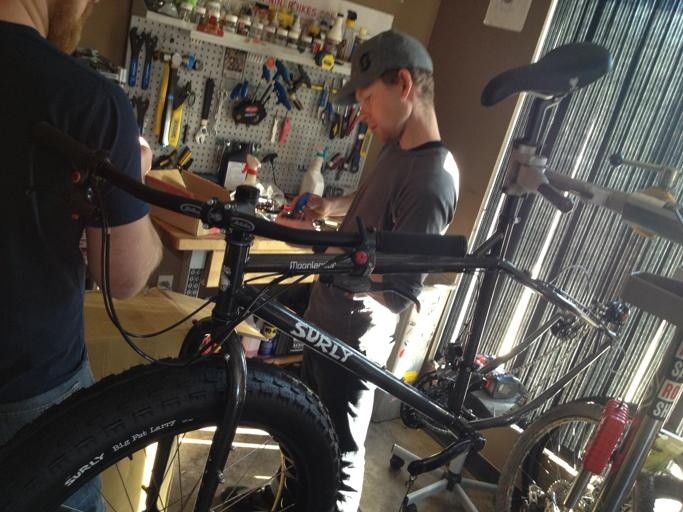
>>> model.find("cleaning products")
[296,145,332,200]
[230,152,262,203]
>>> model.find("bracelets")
[311,219,325,232]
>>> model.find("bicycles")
[0,37,639,510]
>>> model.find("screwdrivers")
[324,79,350,138]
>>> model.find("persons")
[220,28,462,512]
[2,0,158,510]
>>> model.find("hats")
[333,31,433,104]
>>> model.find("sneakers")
[222,484,278,511]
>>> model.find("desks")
[151,216,347,367]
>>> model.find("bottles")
[179,0,368,62]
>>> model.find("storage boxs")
[84,286,269,512]
[144,168,231,238]
